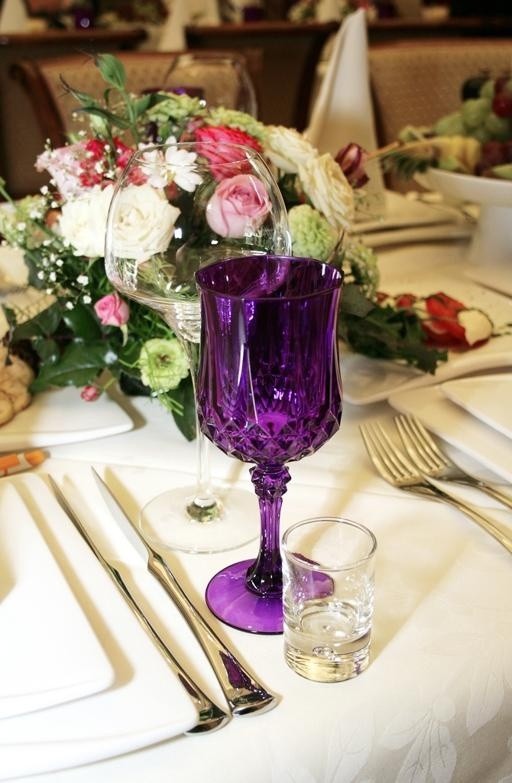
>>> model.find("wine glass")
[192,252,347,637]
[100,139,296,560]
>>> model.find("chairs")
[182,22,341,134]
[298,23,511,147]
[15,45,257,151]
[2,28,148,198]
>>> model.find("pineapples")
[378,135,482,184]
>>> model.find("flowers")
[0,56,499,441]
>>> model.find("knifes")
[90,467,274,716]
[39,469,232,734]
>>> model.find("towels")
[297,7,386,221]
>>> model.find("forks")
[356,407,512,554]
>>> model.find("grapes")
[434,76,512,165]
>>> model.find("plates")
[342,187,474,247]
[386,369,512,490]
[340,275,512,409]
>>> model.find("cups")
[277,514,380,684]
[158,49,261,125]
